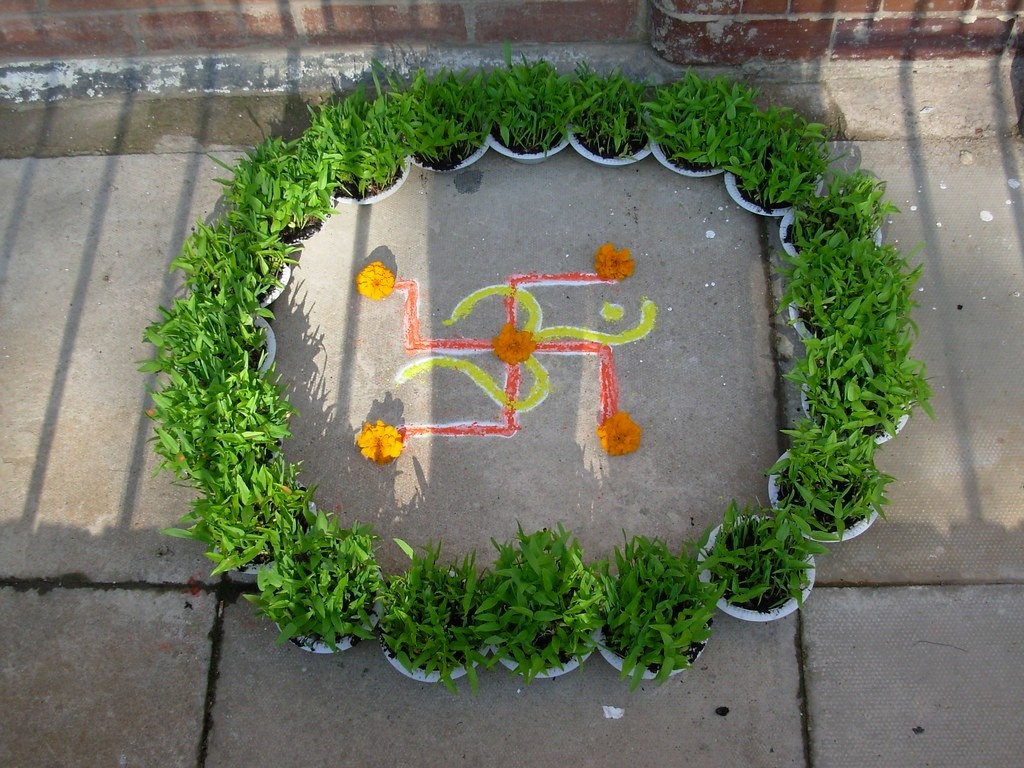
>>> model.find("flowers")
[492,322,538,366]
[593,242,635,283]
[354,418,403,465]
[596,410,640,457]
[354,261,396,302]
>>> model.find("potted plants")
[132,31,935,577]
[694,496,831,624]
[242,502,383,656]
[376,534,489,695]
[594,526,729,693]
[469,518,604,688]
